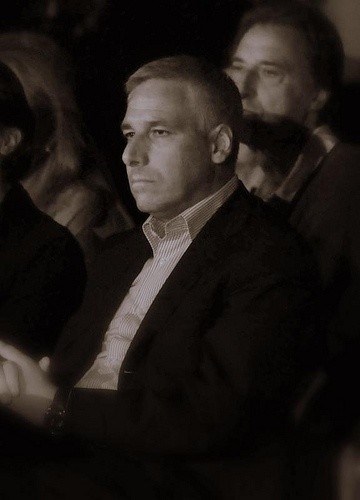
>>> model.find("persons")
[219,6,359,346]
[0,64,85,499]
[0,56,328,500]
[3,30,126,280]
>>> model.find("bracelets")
[41,385,71,436]
